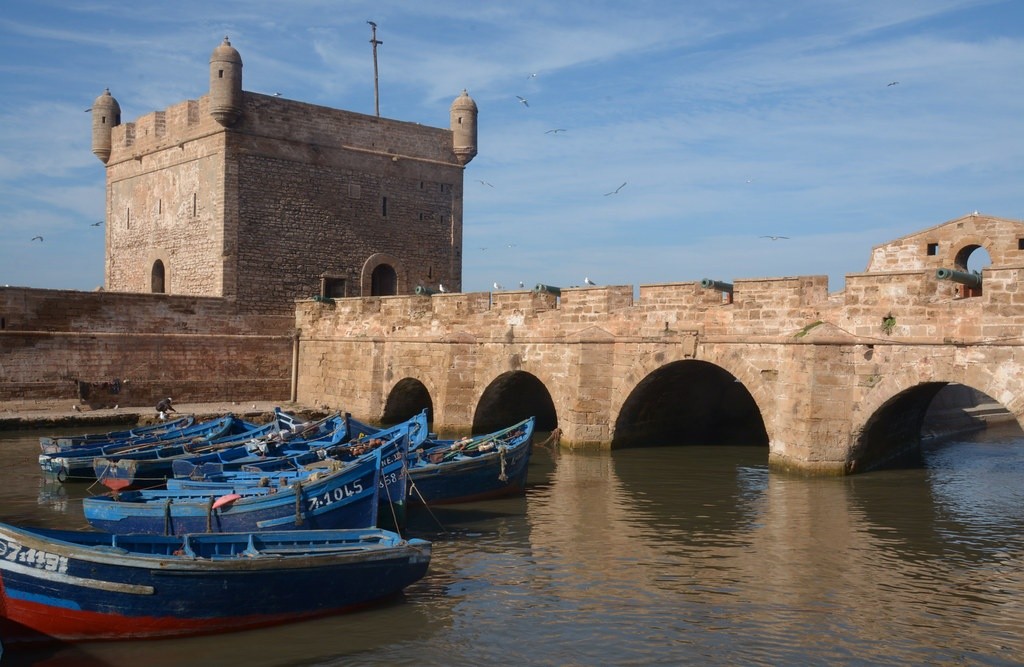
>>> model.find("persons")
[156,397,176,414]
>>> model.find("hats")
[168,398,172,403]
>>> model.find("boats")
[82,448,381,536]
[166,426,410,518]
[37,406,536,499]
[0,520,433,641]
